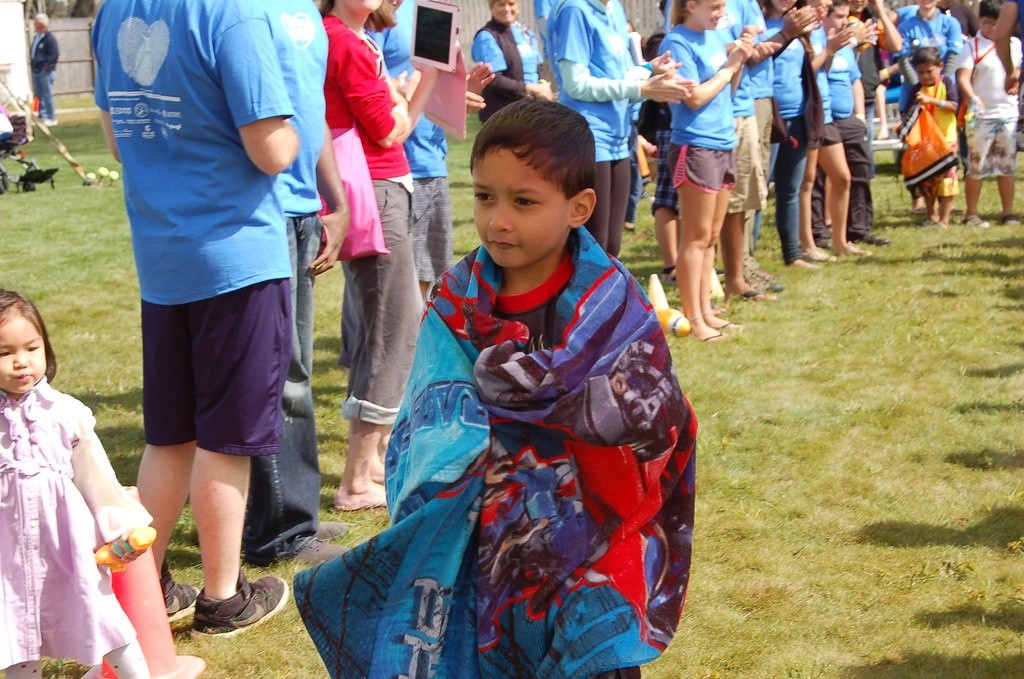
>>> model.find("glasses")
[980,17,996,28]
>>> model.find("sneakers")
[190,570,290,637]
[285,537,351,566]
[314,520,348,537]
[154,559,198,623]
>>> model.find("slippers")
[741,289,776,302]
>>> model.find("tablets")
[410,0,460,72]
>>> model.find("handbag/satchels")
[317,127,391,260]
[898,103,958,190]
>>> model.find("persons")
[92,0,300,638]
[0,288,153,679]
[30,12,59,127]
[317,0,496,511]
[470,0,554,122]
[238,0,351,566]
[294,95,699,679]
[532,0,1024,341]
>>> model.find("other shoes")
[335,482,388,511]
[920,218,947,229]
[641,190,655,202]
[661,264,677,280]
[800,247,837,263]
[961,214,990,229]
[790,259,817,270]
[687,315,726,342]
[704,314,740,333]
[1000,213,1020,225]
[837,241,869,258]
[746,270,781,292]
[625,221,635,232]
[852,231,890,248]
[43,119,59,127]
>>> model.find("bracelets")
[939,100,946,109]
[929,98,934,103]
[967,96,979,105]
[639,62,654,76]
[718,70,732,82]
[849,37,859,47]
[882,67,889,80]
[779,31,788,43]
[530,84,536,92]
